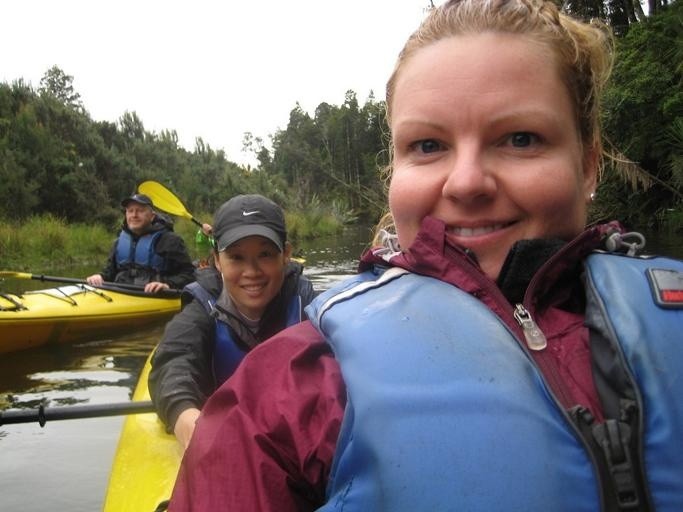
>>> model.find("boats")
[0,253,307,355]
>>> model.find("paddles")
[137,180,213,233]
[0,270,182,296]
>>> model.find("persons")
[164,0,682,512]
[84,192,196,297]
[146,191,332,453]
[195,221,215,260]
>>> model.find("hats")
[211,192,286,255]
[119,193,153,208]
